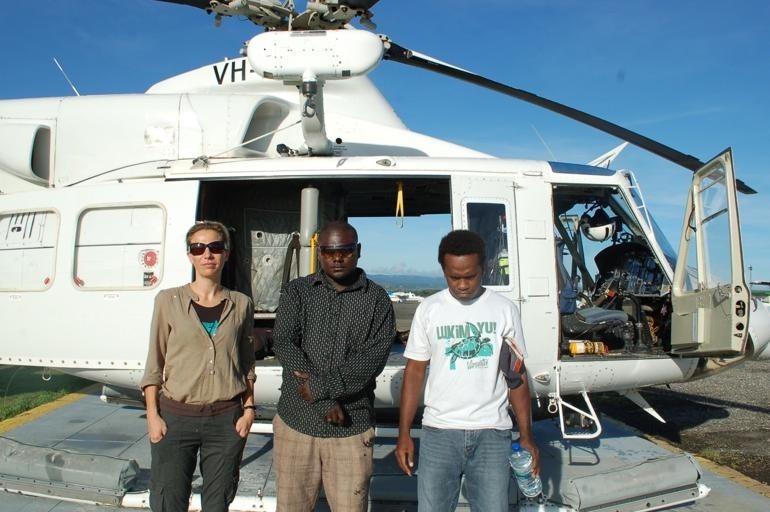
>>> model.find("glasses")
[190,241,225,255]
[319,248,355,258]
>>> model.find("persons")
[394,229,541,512]
[141,219,256,512]
[273,222,396,512]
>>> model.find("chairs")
[553,237,633,356]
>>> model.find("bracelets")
[243,405,256,410]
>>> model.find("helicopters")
[1,0,769,443]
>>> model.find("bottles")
[569,340,609,355]
[507,441,543,498]
[498,248,510,276]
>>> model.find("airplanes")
[388,291,427,303]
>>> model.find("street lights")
[748,261,753,286]
